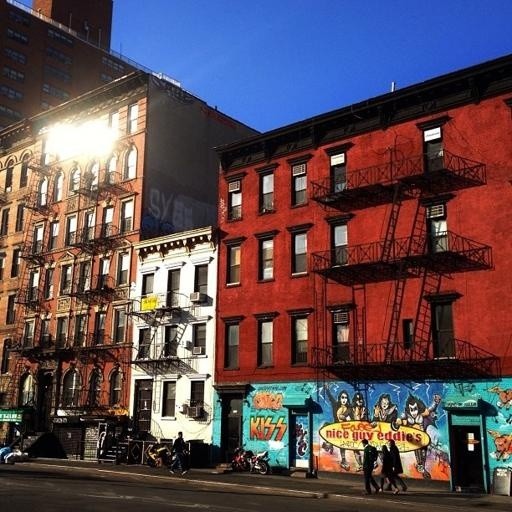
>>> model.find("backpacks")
[366,446,378,461]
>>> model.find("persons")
[377,444,399,495]
[350,391,377,470]
[373,392,401,432]
[323,382,353,470]
[386,440,408,492]
[168,430,189,475]
[393,391,442,473]
[362,439,382,496]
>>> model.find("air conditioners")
[185,406,201,418]
[192,346,205,355]
[189,292,207,303]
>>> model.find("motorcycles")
[232,444,271,475]
[145,441,170,468]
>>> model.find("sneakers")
[364,485,408,495]
[169,470,187,476]
